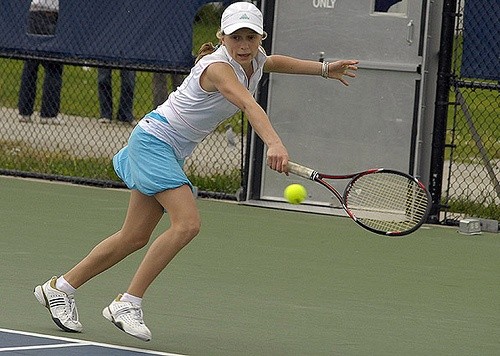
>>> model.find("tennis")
[284,184,307,205]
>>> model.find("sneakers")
[102,293,152,343]
[33,275,83,334]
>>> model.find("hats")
[220,1,264,36]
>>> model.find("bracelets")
[321,60,329,79]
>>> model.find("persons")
[32,1,359,342]
